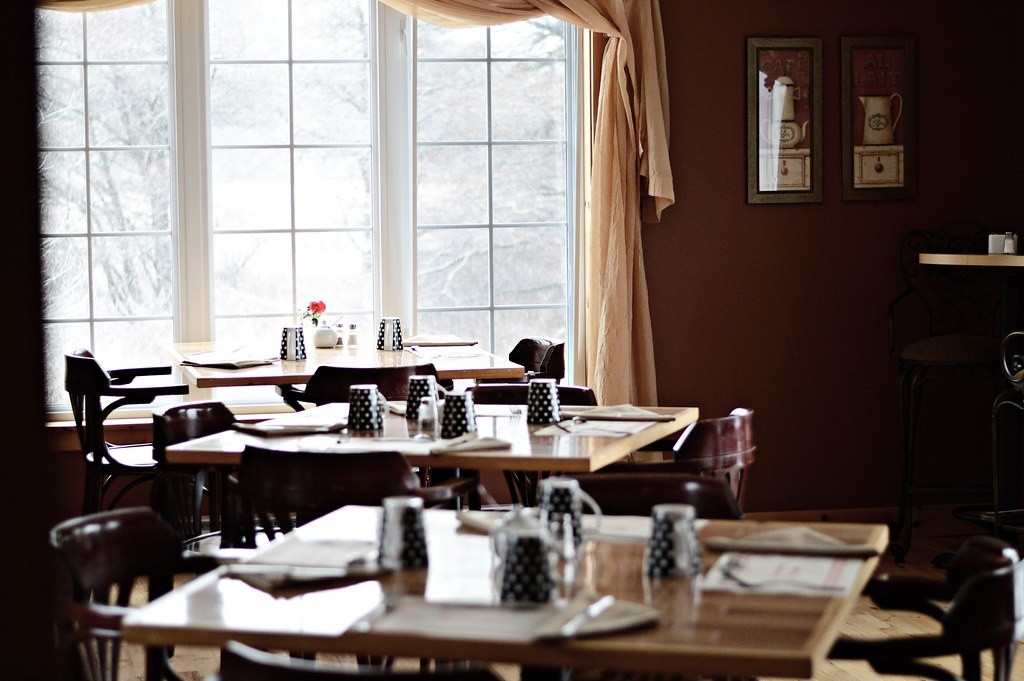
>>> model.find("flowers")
[302,300,326,325]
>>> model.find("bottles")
[348,324,357,349]
[1003,232,1014,254]
[335,324,344,347]
[419,397,438,435]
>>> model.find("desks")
[173,337,525,412]
[164,400,701,515]
[120,502,891,678]
[918,252,1024,538]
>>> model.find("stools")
[883,220,1019,566]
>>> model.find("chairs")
[46,335,1021,681]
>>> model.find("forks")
[554,422,630,434]
[509,408,522,413]
[721,568,843,590]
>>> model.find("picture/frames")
[839,35,919,202]
[745,37,824,205]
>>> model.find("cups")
[377,497,429,571]
[526,378,560,423]
[646,504,702,580]
[406,375,448,420]
[988,235,1017,254]
[537,478,601,545]
[377,317,403,351]
[494,529,581,607]
[442,392,476,439]
[347,384,389,431]
[280,325,306,361]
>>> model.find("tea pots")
[313,320,338,348]
